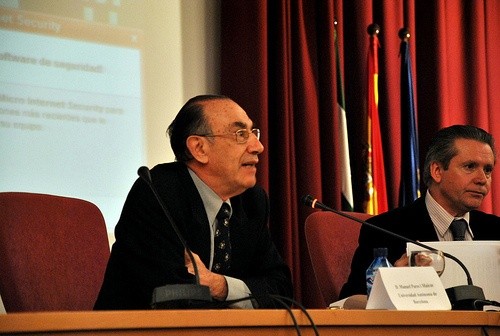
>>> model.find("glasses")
[198,129,260,143]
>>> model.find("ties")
[211,202,233,276]
[449,219,467,241]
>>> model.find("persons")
[93,94,298,311]
[339,124,500,300]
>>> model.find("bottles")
[366,247,395,297]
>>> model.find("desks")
[0,309,500,336]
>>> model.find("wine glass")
[410,250,445,277]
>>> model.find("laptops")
[406,240,500,303]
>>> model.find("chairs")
[305,211,376,308]
[0,193,110,313]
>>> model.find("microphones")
[301,194,485,310]
[137,166,212,309]
